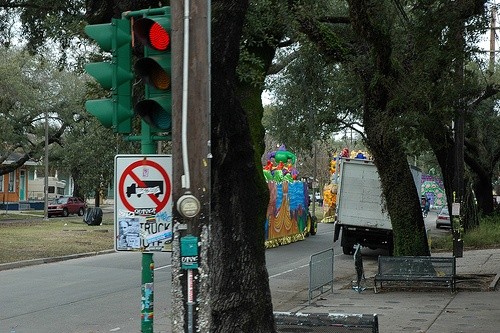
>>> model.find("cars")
[48,196,87,216]
[436,206,451,228]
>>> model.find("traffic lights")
[150,22,171,130]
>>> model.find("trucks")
[334,156,422,259]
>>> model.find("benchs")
[373,254,456,295]
